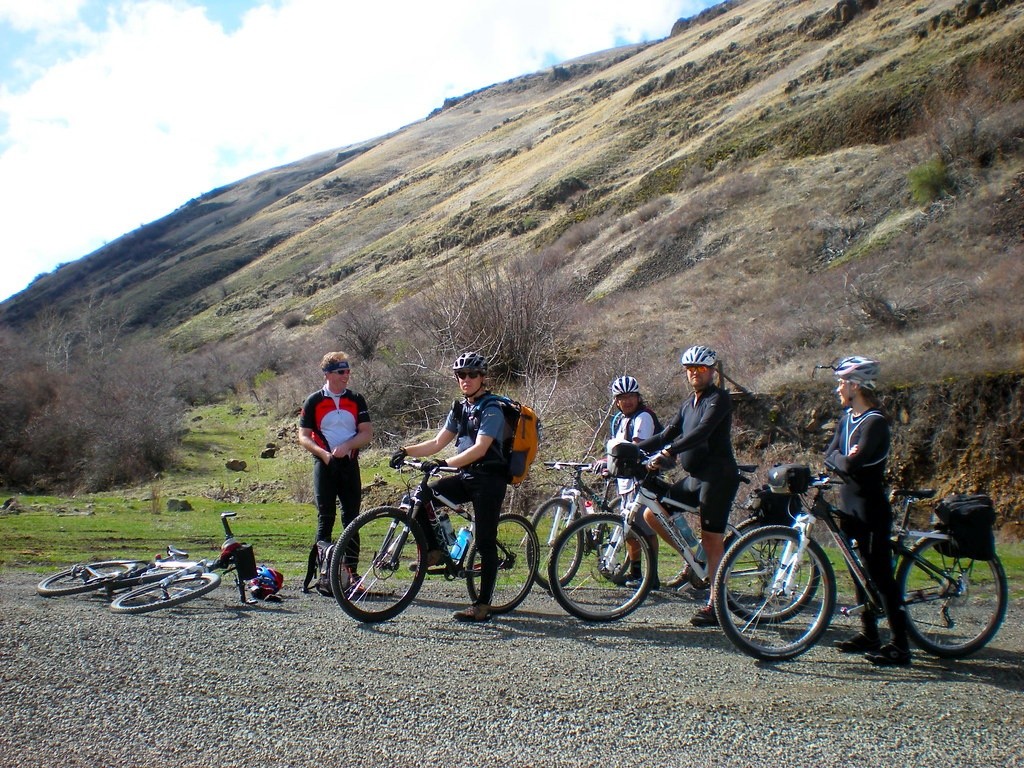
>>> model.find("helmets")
[245,566,283,598]
[835,356,881,389]
[453,352,488,374]
[611,376,639,397]
[681,345,718,368]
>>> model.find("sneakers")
[866,644,911,668]
[407,551,443,570]
[452,604,492,622]
[833,633,882,653]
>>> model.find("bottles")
[851,538,864,568]
[585,500,595,515]
[672,511,698,547]
[439,509,458,546]
[450,526,471,560]
[695,542,706,561]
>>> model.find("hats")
[322,360,350,374]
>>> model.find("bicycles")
[329,458,540,624]
[527,462,635,589]
[713,459,1008,661]
[37,512,258,614]
[547,438,821,623]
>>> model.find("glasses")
[456,372,480,379]
[685,366,709,373]
[331,370,351,374]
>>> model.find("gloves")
[420,459,448,475]
[646,453,669,471]
[389,448,407,469]
[593,459,607,474]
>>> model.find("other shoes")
[666,568,688,587]
[613,561,643,584]
[692,605,719,627]
[349,576,365,591]
[626,576,661,591]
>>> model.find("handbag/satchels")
[932,495,995,561]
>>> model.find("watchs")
[664,444,673,453]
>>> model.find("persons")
[390,352,507,620]
[592,374,661,589]
[298,352,372,593]
[637,346,740,627]
[823,356,911,666]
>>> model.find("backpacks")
[457,395,541,487]
[302,539,351,598]
